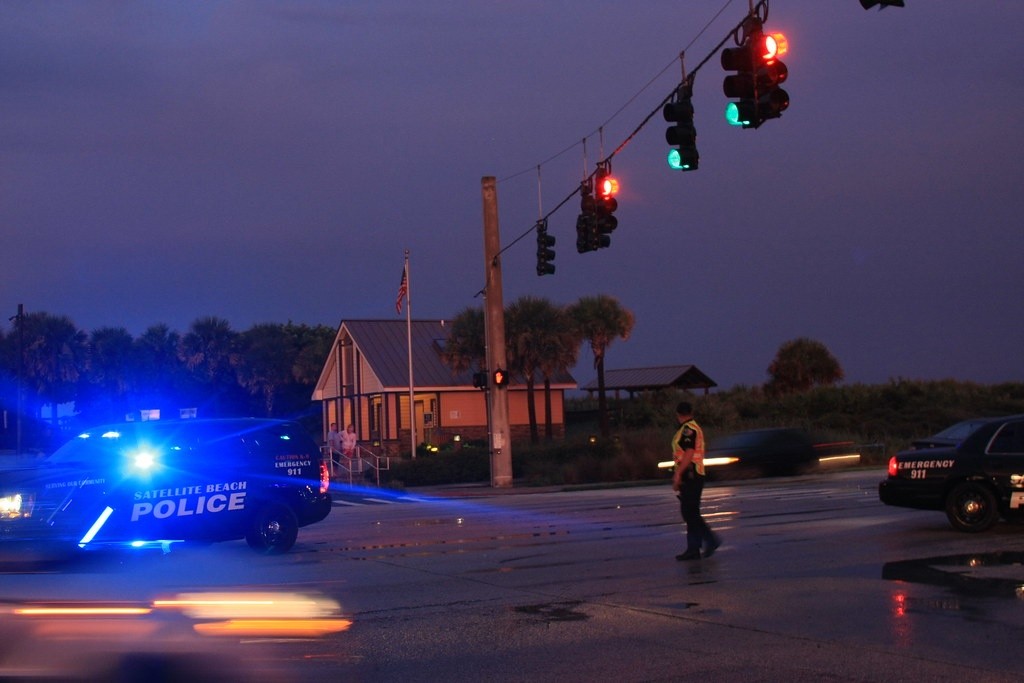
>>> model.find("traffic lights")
[596,164,618,248]
[577,193,597,253]
[751,31,788,118]
[721,42,767,129]
[536,225,555,276]
[662,89,699,172]
[494,368,510,386]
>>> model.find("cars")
[656,426,817,484]
[877,415,1024,535]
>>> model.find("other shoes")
[701,538,722,558]
[675,547,701,560]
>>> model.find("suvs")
[0,417,332,572]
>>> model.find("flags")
[396,263,407,314]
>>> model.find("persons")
[338,424,356,474]
[672,402,722,560]
[327,423,341,469]
